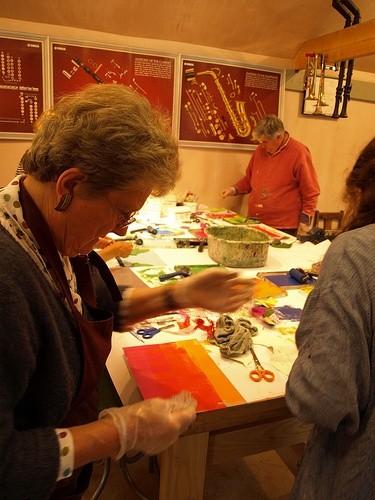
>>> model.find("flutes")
[330,0,351,119]
[341,0,365,121]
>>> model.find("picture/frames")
[0,30,45,140]
[176,53,286,151]
[46,35,178,143]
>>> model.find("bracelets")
[164,280,177,310]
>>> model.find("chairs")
[314,210,344,230]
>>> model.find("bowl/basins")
[202,225,274,268]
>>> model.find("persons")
[219,113,320,243]
[283,137,375,500]
[0,83,257,500]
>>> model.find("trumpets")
[311,71,324,116]
[305,54,317,101]
[316,58,329,107]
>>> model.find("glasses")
[104,193,137,230]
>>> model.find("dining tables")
[95,203,323,500]
